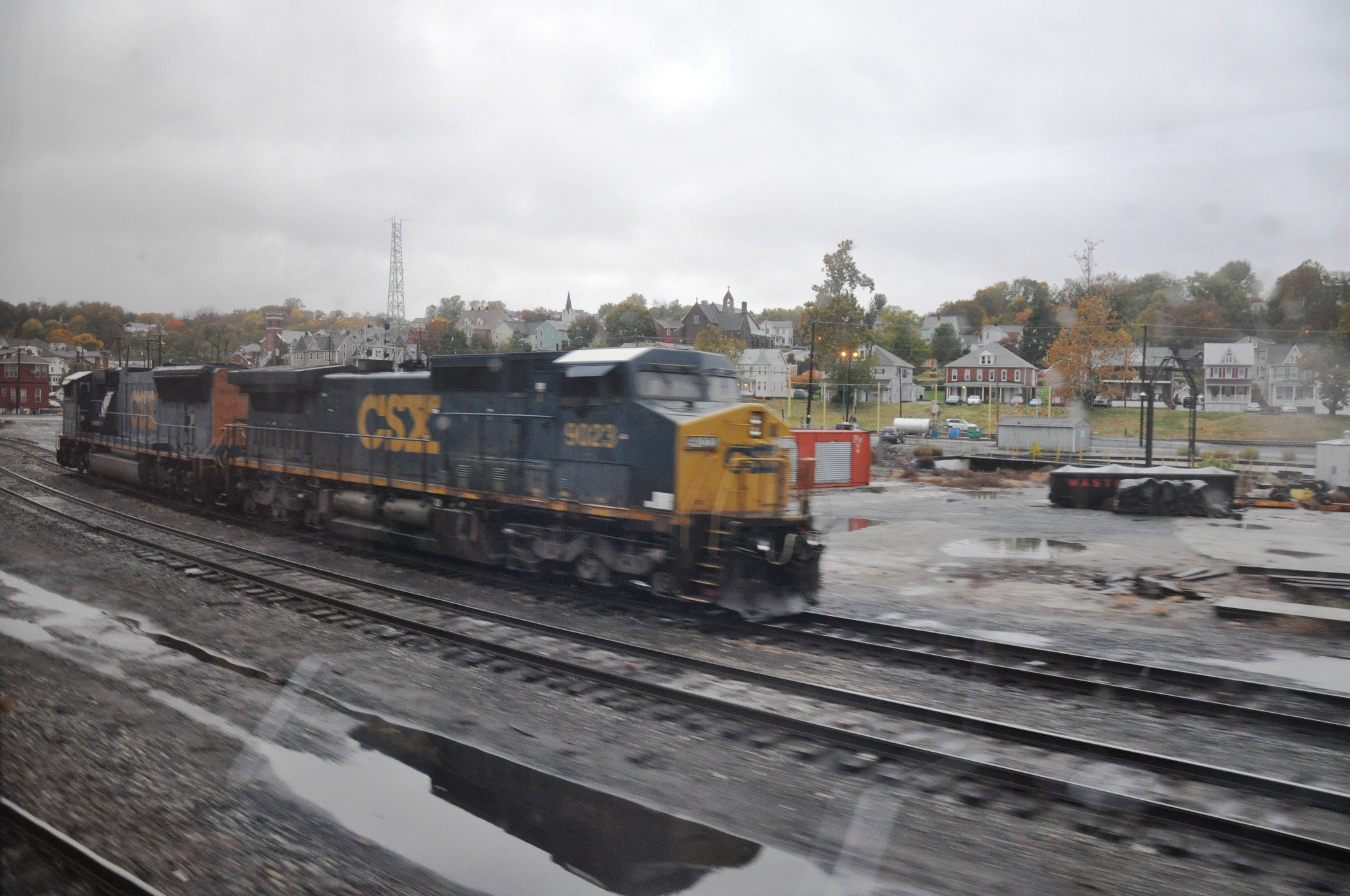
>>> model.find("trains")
[57,347,821,622]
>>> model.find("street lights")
[841,347,858,421]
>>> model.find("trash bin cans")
[835,422,853,431]
[967,427,983,438]
[951,427,961,438]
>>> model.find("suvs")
[1182,397,1197,408]
[945,418,979,432]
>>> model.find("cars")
[1245,402,1262,413]
[946,394,1113,408]
[1281,403,1297,413]
